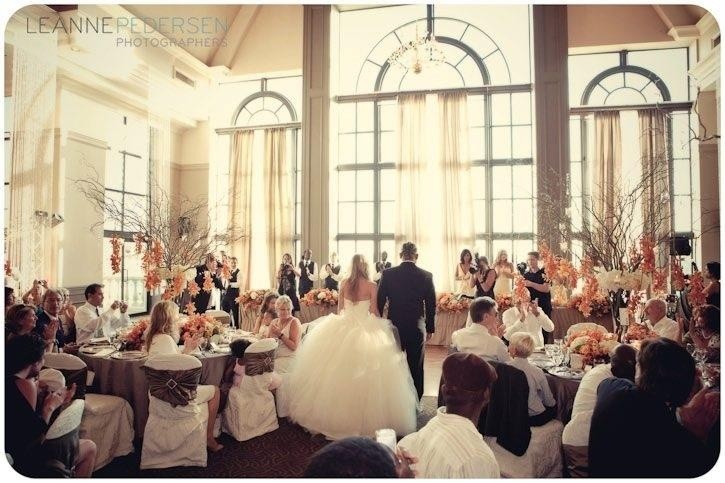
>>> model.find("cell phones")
[375,429,398,450]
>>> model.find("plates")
[82,347,115,354]
[214,347,231,353]
[88,341,110,345]
[110,351,145,362]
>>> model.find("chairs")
[476,360,564,478]
[206,310,230,327]
[41,353,135,471]
[276,323,313,423]
[140,354,209,471]
[222,338,279,442]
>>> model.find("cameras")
[36,280,49,288]
[468,253,482,275]
[280,264,288,271]
[216,261,223,268]
[516,262,528,272]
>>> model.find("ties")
[306,262,308,267]
[332,263,334,268]
[96,308,100,317]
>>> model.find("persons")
[285,254,420,441]
[395,353,501,478]
[375,251,391,285]
[302,435,416,478]
[452,243,720,479]
[5,250,342,478]
[377,242,436,413]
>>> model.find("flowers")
[177,313,226,345]
[495,292,515,312]
[437,294,470,314]
[567,323,618,369]
[566,292,609,319]
[625,325,659,349]
[301,289,339,312]
[235,289,269,313]
[117,319,149,351]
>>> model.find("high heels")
[211,444,223,452]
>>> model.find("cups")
[545,337,565,369]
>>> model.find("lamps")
[385,22,446,74]
